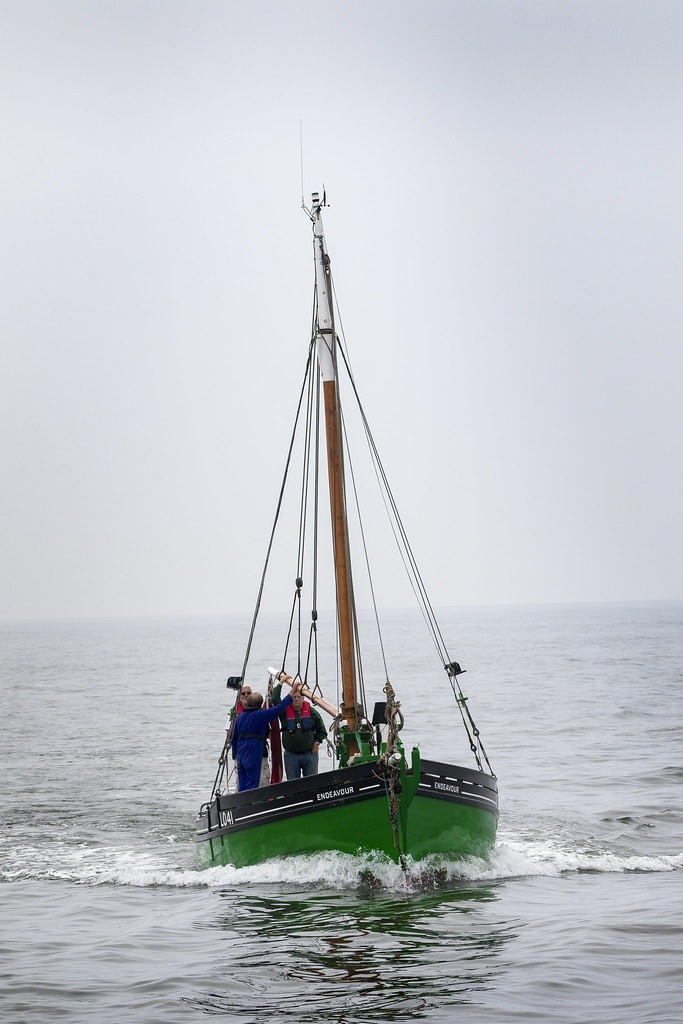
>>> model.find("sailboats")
[196,187,499,878]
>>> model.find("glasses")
[240,692,251,696]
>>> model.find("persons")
[232,684,304,792]
[270,676,327,781]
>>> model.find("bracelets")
[315,740,319,744]
